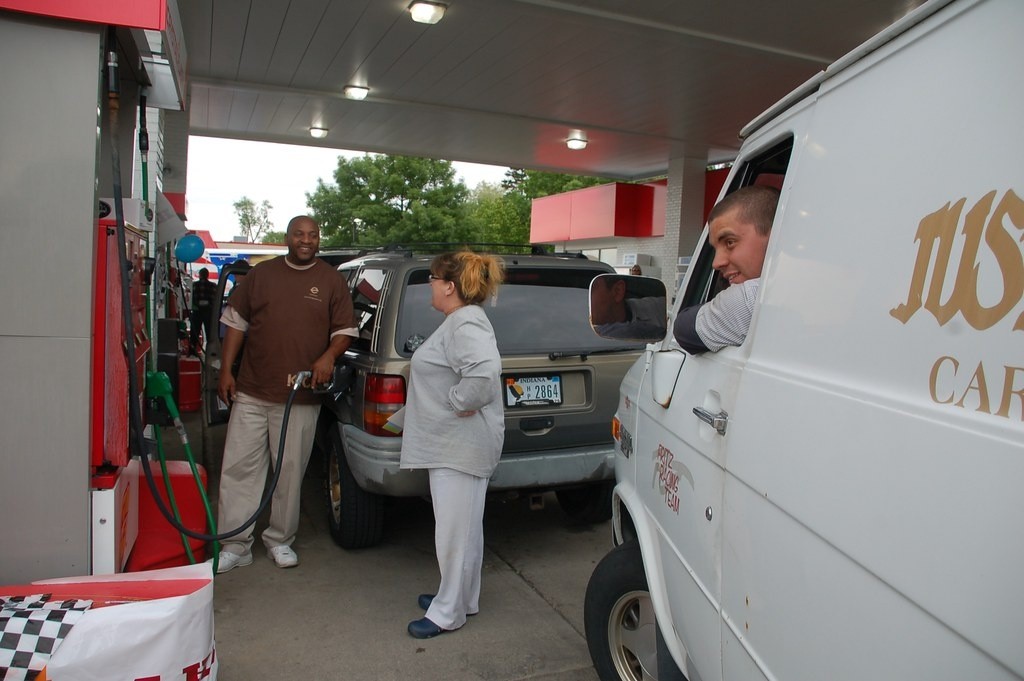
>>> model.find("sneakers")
[266,545,299,568]
[202,551,253,573]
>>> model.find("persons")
[204,216,360,573]
[591,275,668,340]
[631,265,642,275]
[188,266,217,350]
[670,181,781,355]
[398,251,505,638]
[227,260,250,298]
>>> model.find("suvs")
[203,243,646,551]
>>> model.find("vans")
[583,0,1024,681]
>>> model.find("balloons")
[174,234,205,263]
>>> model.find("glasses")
[428,275,449,286]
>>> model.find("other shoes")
[408,616,443,638]
[419,593,434,609]
